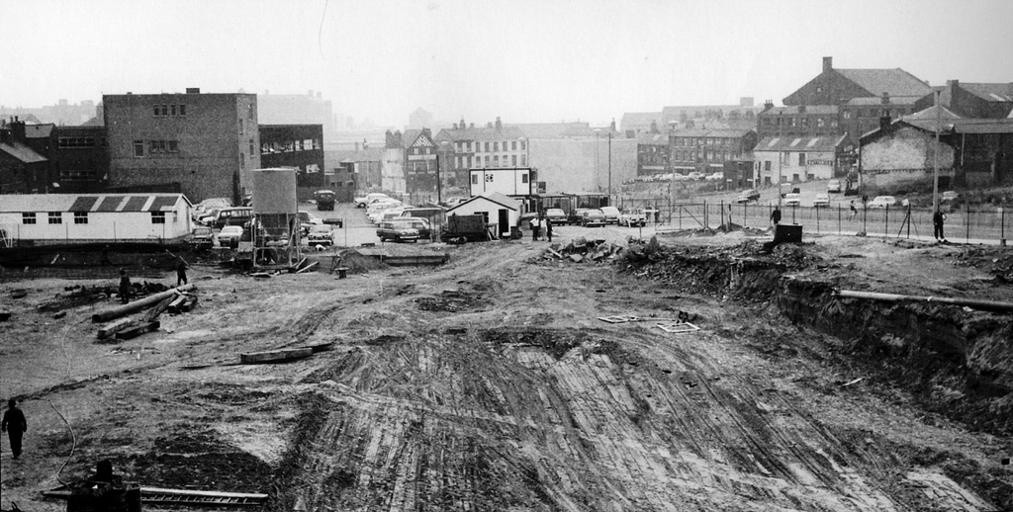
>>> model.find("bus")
[313,190,336,210]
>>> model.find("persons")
[119,266,130,304]
[177,261,188,285]
[934,206,944,239]
[530,215,555,242]
[770,205,782,225]
[2,398,28,460]
[850,200,858,214]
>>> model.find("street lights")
[594,129,602,164]
[776,106,789,210]
[668,121,678,223]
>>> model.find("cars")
[544,206,646,227]
[301,211,343,246]
[940,191,959,205]
[738,189,760,203]
[781,180,896,210]
[190,207,253,248]
[637,172,722,182]
[353,193,439,242]
